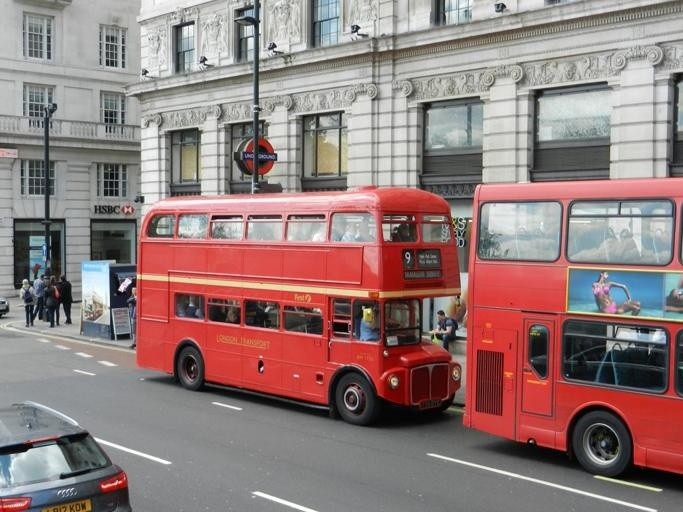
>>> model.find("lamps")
[351,25,369,38]
[268,42,283,54]
[141,69,154,78]
[493,2,507,13]
[199,57,215,67]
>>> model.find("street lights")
[41,103,57,279]
[235,15,260,196]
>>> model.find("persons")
[248,216,418,244]
[663,276,682,312]
[31,272,45,321]
[426,308,456,351]
[19,278,35,327]
[43,274,59,328]
[357,306,379,342]
[589,270,641,317]
[125,286,137,349]
[184,295,203,319]
[56,274,72,324]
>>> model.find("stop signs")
[241,137,277,174]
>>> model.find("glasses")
[675,291,682,300]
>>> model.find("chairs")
[515,225,668,265]
[530,329,683,394]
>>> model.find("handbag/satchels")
[54,289,60,299]
[431,330,442,346]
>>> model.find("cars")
[0,296,9,318]
[0,401,133,511]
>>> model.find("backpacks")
[22,287,33,303]
[444,317,457,329]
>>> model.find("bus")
[134,186,460,426]
[465,177,683,479]
